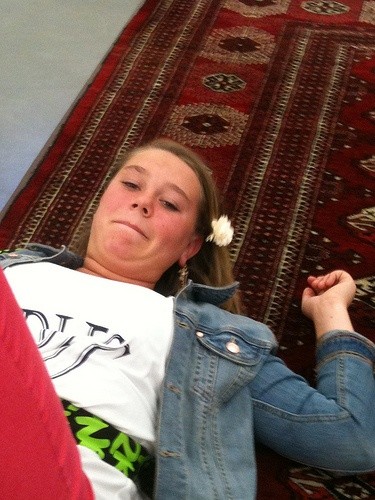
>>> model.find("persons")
[1,135,375,500]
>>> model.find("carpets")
[0,0,375,500]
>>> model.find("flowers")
[206,213,234,246]
